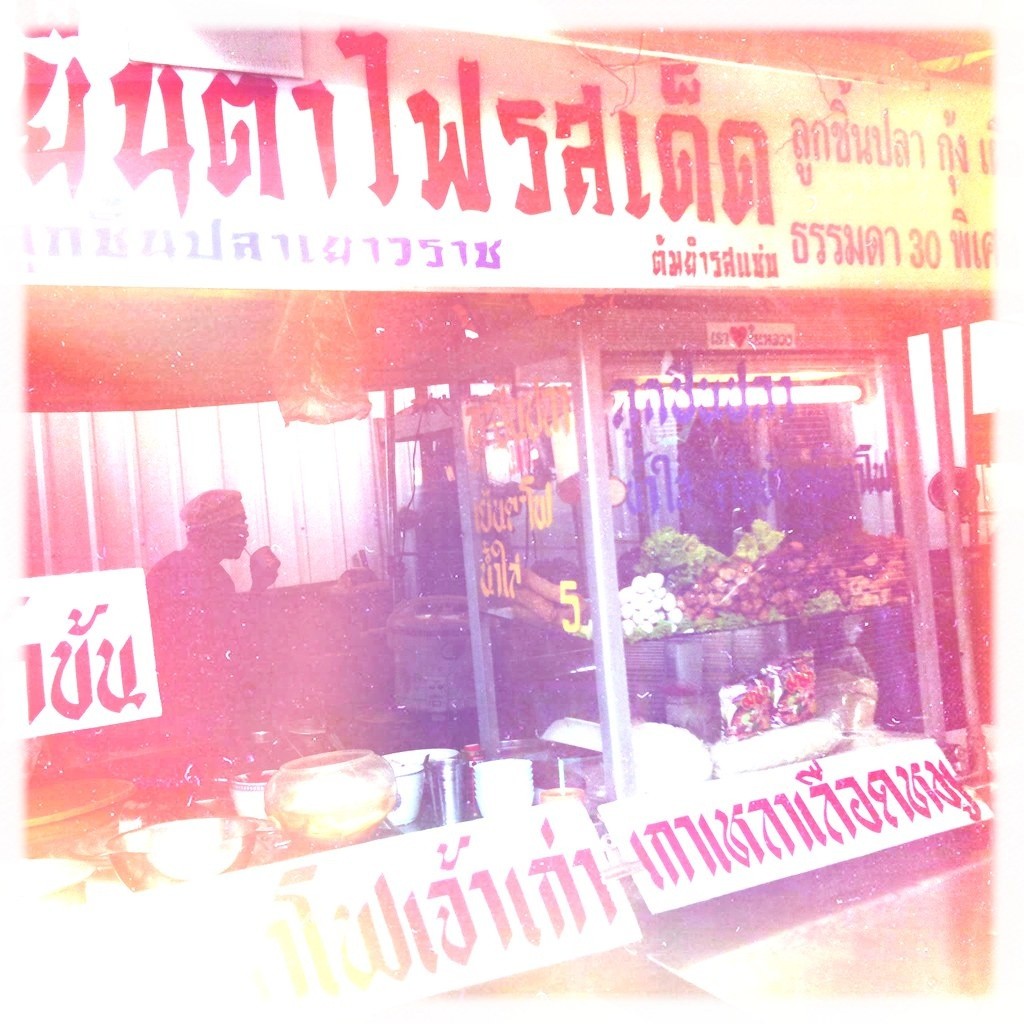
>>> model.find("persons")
[143,488,281,731]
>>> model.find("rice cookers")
[387,595,476,713]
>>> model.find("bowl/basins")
[105,816,259,893]
[541,717,602,752]
[473,759,535,818]
[26,777,137,859]
[384,762,425,827]
[230,767,279,820]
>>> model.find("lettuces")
[619,518,843,643]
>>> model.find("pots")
[264,748,398,843]
[384,749,464,833]
